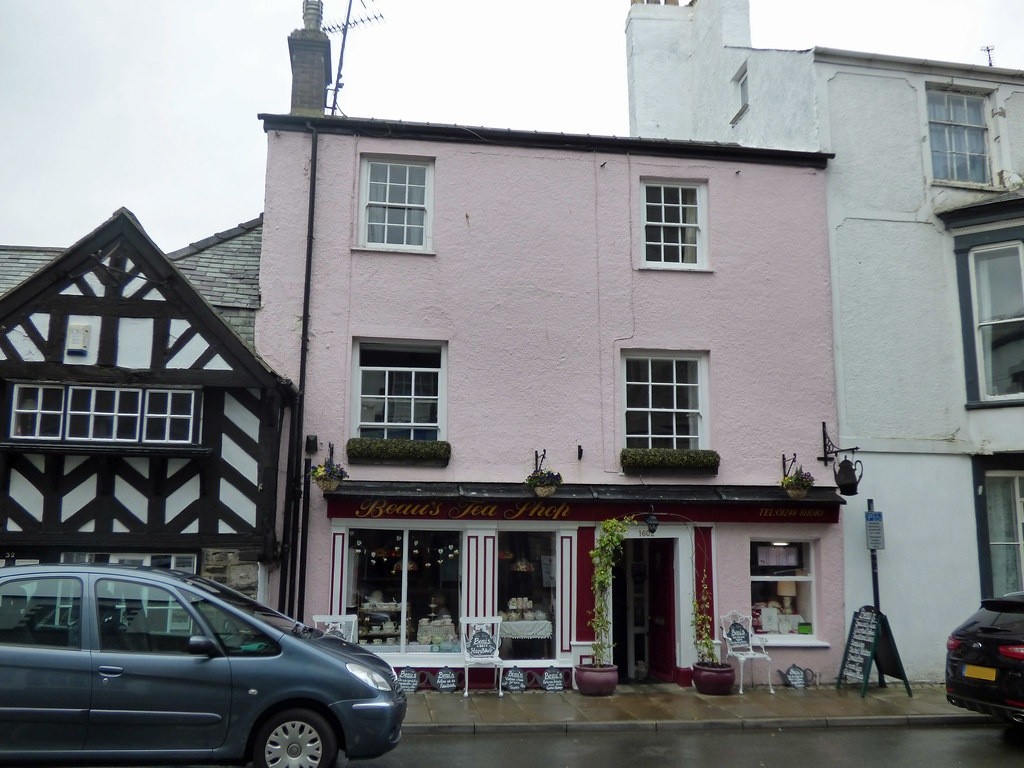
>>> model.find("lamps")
[645,504,660,534]
[778,581,797,615]
[817,421,864,497]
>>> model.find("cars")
[0,561,406,768]
[946,591,1024,727]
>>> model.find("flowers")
[306,457,350,481]
[521,466,563,486]
[780,464,817,486]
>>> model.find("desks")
[502,621,552,658]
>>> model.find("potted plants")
[690,569,737,696]
[574,514,638,696]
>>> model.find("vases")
[535,486,557,498]
[786,486,807,497]
[316,480,339,491]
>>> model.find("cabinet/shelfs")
[359,606,411,642]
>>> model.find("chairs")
[721,609,776,695]
